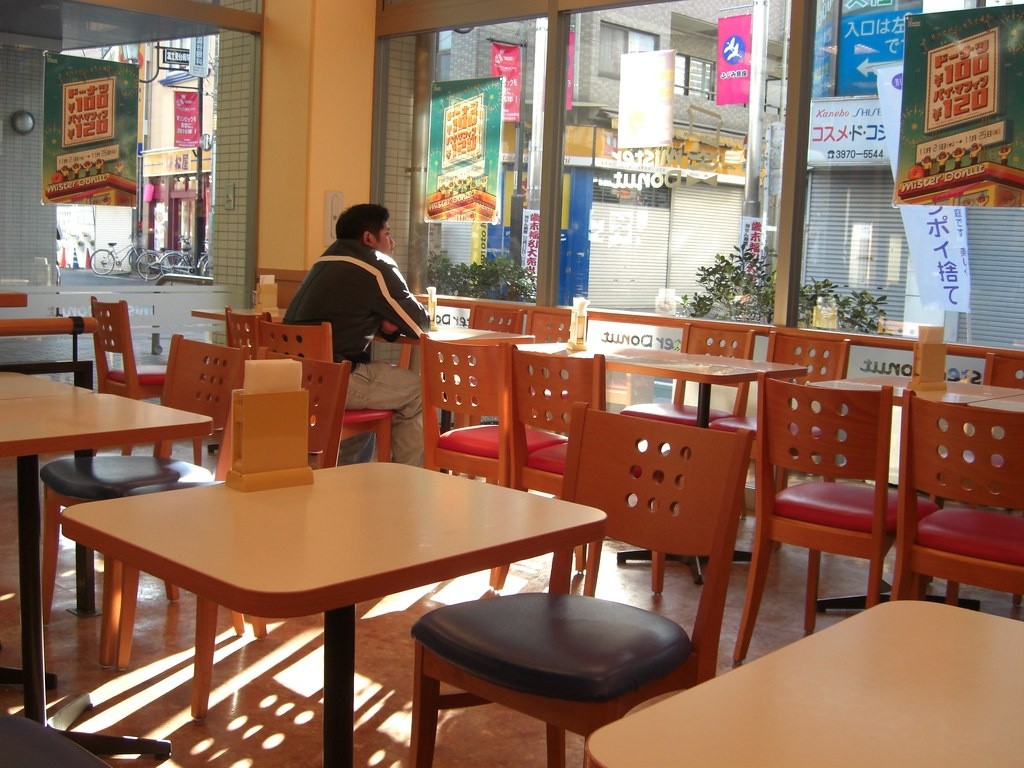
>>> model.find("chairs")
[731,369,939,662]
[422,334,588,586]
[526,307,589,401]
[931,352,1024,511]
[452,305,524,481]
[621,322,756,519]
[41,295,393,671]
[710,330,852,551]
[493,345,674,595]
[408,401,757,768]
[892,391,1024,604]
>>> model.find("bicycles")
[89,236,149,275]
[136,236,209,281]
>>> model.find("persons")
[281,205,430,469]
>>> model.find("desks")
[801,373,1024,613]
[191,305,809,588]
[60,463,609,768]
[0,372,215,760]
[585,601,1024,768]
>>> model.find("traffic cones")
[60,246,67,268]
[86,247,91,270]
[72,248,84,269]
